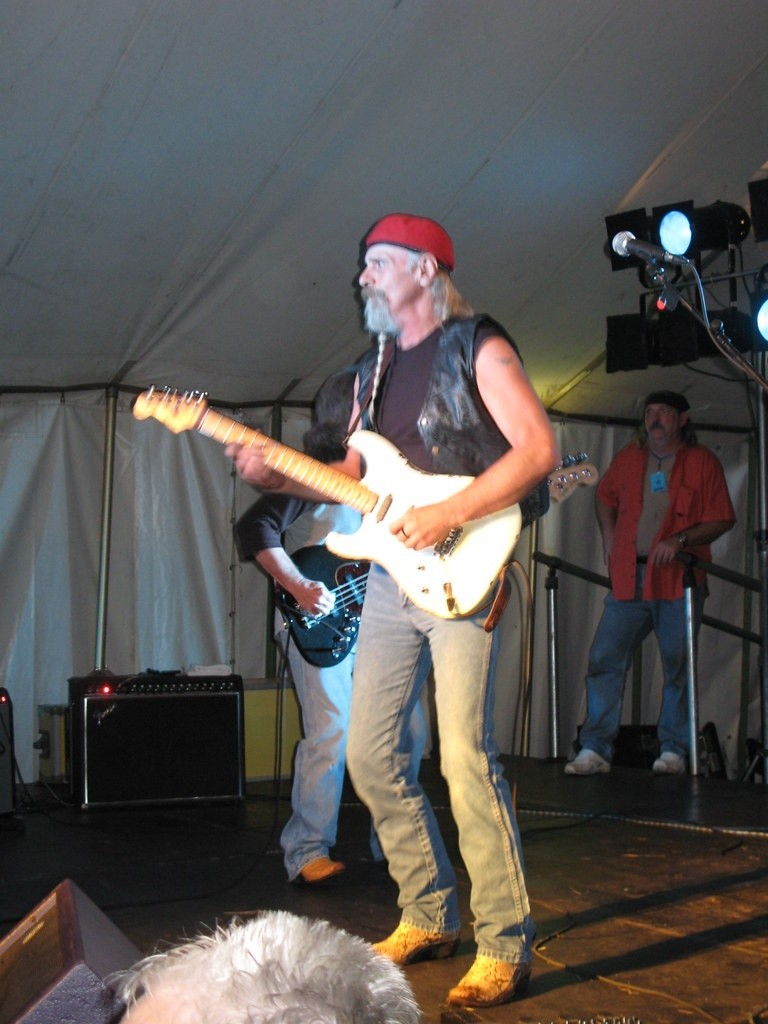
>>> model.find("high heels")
[371,921,463,966]
[446,955,532,1007]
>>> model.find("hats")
[644,390,691,414]
[365,214,457,273]
[316,370,357,423]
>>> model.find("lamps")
[605,178,768,373]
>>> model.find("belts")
[636,555,648,564]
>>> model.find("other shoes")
[380,859,388,865]
[301,856,345,883]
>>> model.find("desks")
[241,677,304,782]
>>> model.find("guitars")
[272,450,599,669]
[132,384,522,620]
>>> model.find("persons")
[565,389,736,776]
[233,368,428,882]
[225,213,561,1006]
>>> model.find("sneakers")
[564,748,611,774]
[653,751,686,773]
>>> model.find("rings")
[402,528,408,538]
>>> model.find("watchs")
[677,532,687,546]
